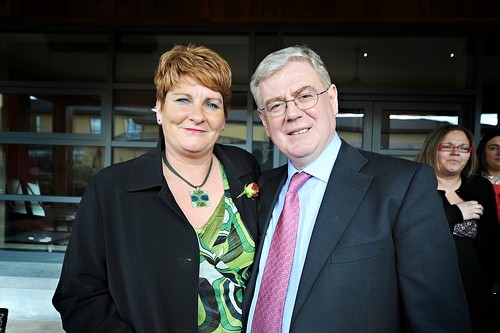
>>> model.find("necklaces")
[163,152,214,208]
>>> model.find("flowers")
[236,183,260,200]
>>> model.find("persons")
[240,46,466,333]
[419,124,500,333]
[52,43,261,333]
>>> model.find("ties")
[251,171,313,333]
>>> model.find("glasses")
[259,88,328,117]
[437,143,474,153]
